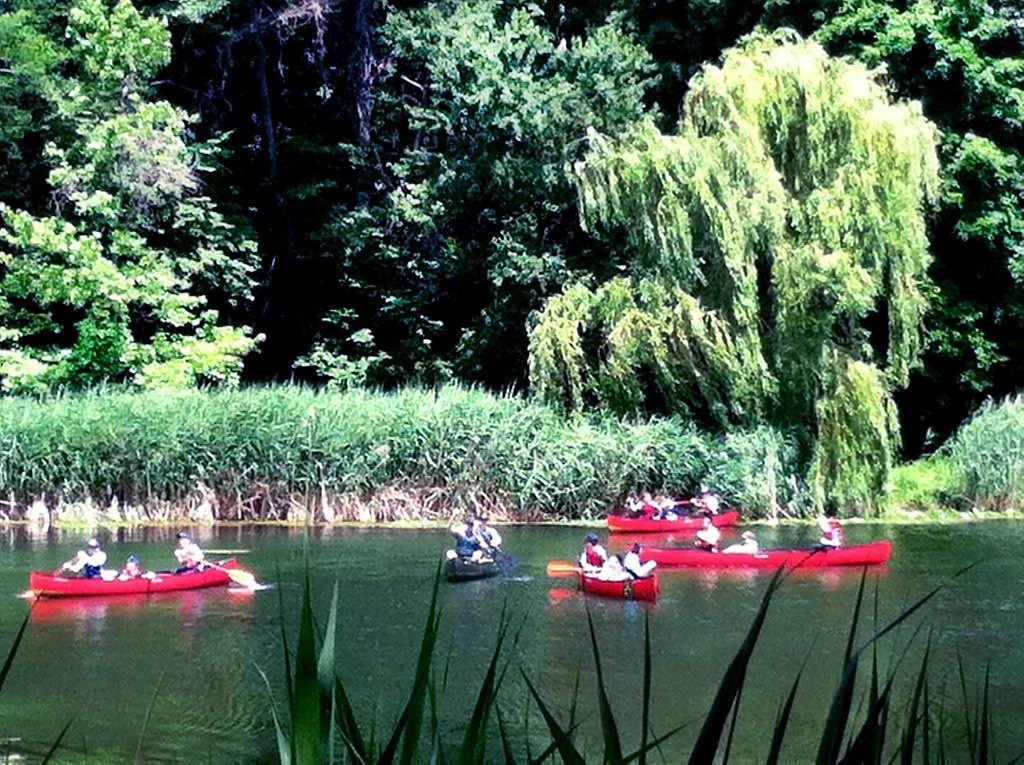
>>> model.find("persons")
[123,555,150,577]
[446,512,502,565]
[580,532,608,578]
[624,489,719,522]
[816,507,843,553]
[173,532,204,576]
[62,539,107,579]
[694,517,721,553]
[597,542,657,582]
[721,531,758,554]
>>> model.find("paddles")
[190,556,255,586]
[51,555,79,576]
[546,559,603,578]
[456,530,517,575]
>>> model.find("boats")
[577,562,662,603]
[630,536,891,569]
[28,556,246,600]
[442,546,504,581]
[604,509,742,533]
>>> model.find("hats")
[88,539,100,548]
[584,533,597,543]
[126,554,141,566]
[176,533,189,538]
[475,513,489,521]
